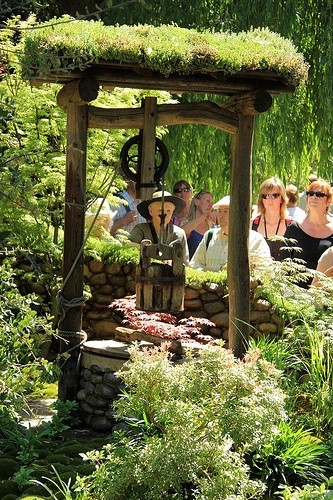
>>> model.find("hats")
[137,191,187,220]
[212,196,230,210]
[85,197,118,218]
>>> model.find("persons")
[181,190,216,260]
[283,184,306,224]
[189,195,272,274]
[310,246,333,307]
[298,174,333,215]
[106,178,150,239]
[171,180,194,229]
[83,197,122,243]
[279,180,333,290]
[128,191,190,268]
[252,178,295,260]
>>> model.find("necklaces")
[264,218,281,239]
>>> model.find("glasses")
[173,187,190,193]
[195,189,205,198]
[261,193,281,199]
[306,190,328,198]
